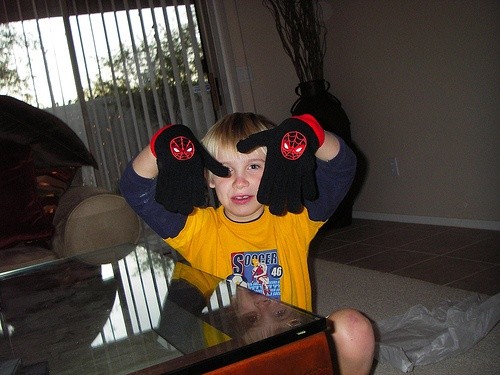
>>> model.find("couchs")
[0,95,143,275]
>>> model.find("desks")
[0,242,336,375]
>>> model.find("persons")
[166,262,300,343]
[119,112,375,375]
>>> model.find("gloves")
[236,113,325,217]
[148,123,230,214]
